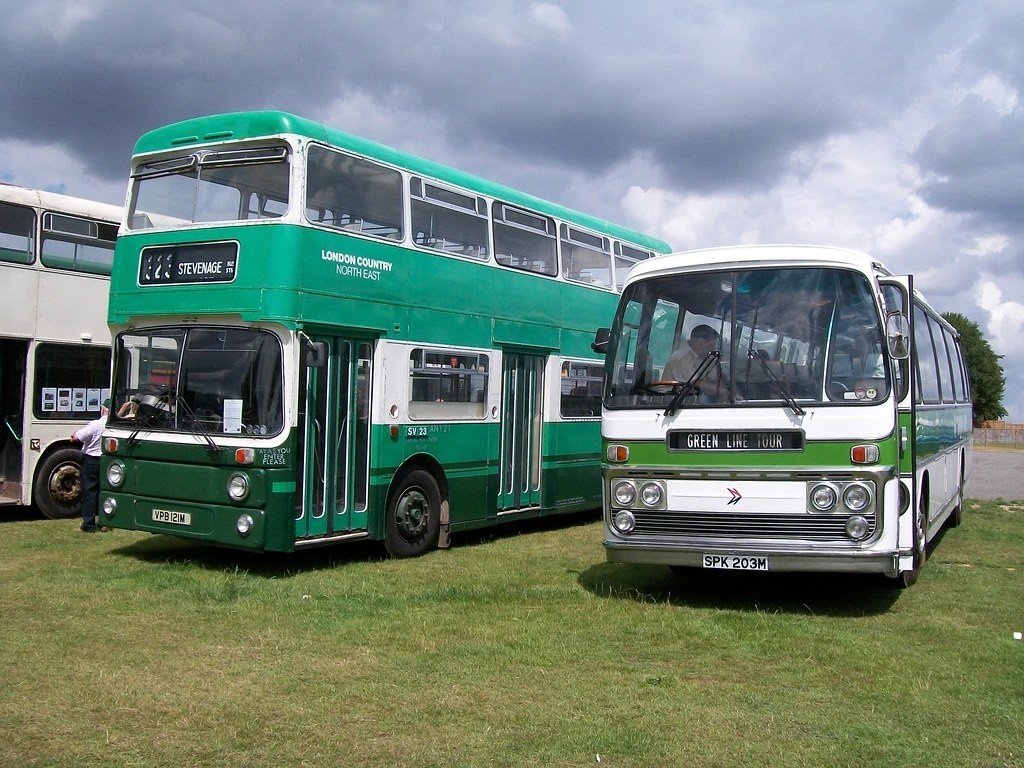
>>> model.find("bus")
[589,243,977,591]
[0,184,197,521]
[94,110,673,558]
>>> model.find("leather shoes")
[80,524,107,533]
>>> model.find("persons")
[658,322,746,404]
[70,397,112,533]
[753,349,771,361]
[116,393,144,422]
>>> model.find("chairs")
[724,355,815,399]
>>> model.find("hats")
[100,398,111,410]
